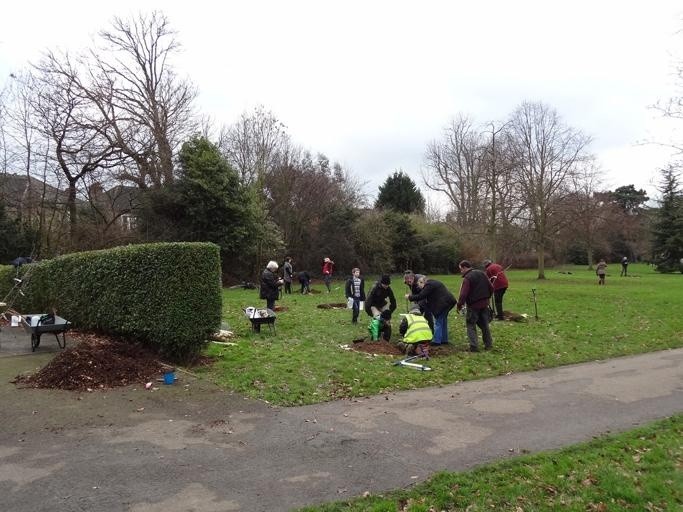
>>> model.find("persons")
[282,255,293,294]
[595,259,607,285]
[455,259,494,352]
[258,259,285,312]
[321,255,335,294]
[483,259,508,319]
[344,267,366,325]
[363,268,457,359]
[296,268,311,296]
[619,256,629,277]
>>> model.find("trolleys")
[241,306,277,337]
[16,307,80,351]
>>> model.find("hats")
[408,301,420,311]
[381,275,390,285]
[382,310,391,319]
[414,274,423,284]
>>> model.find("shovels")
[532,289,538,321]
